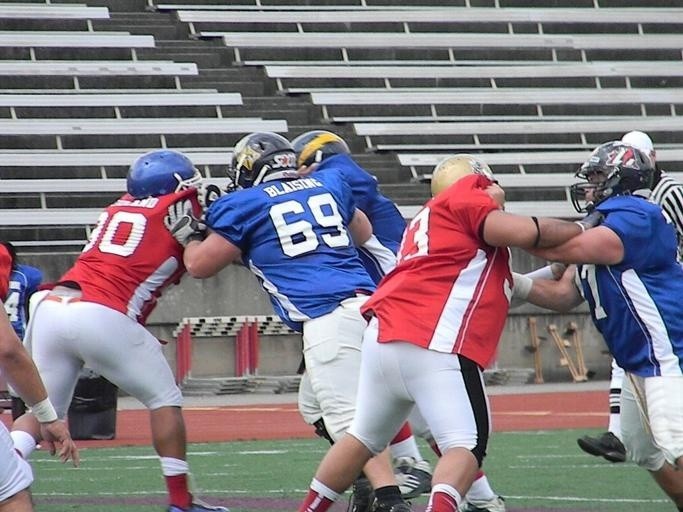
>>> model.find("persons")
[197,132,414,512]
[17,150,228,512]
[0,240,79,510]
[577,130,683,463]
[288,128,504,512]
[511,141,683,510]
[3,245,43,421]
[293,154,605,511]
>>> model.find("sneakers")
[460,495,505,512]
[345,479,378,511]
[577,432,626,462]
[166,492,228,512]
[392,455,432,499]
[374,501,412,511]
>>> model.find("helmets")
[126,149,202,206]
[569,141,655,214]
[430,153,498,199]
[290,130,350,176]
[225,132,299,192]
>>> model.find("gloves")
[573,210,605,232]
[162,200,207,248]
[196,185,220,208]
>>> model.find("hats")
[620,130,654,157]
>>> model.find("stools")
[528,316,589,385]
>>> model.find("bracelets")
[31,396,57,424]
[574,219,586,231]
[508,272,533,300]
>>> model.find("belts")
[40,295,79,304]
